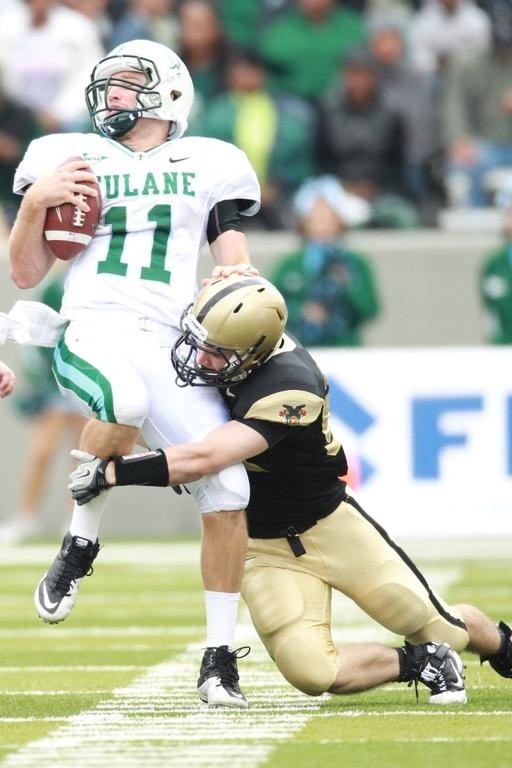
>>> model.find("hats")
[293,175,374,226]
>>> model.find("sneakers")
[34,530,99,625]
[197,646,246,708]
[409,641,468,706]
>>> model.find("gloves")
[69,449,112,507]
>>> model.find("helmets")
[169,273,288,387]
[85,38,194,142]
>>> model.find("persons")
[0,361,16,399]
[14,270,91,526]
[9,38,264,706]
[68,273,512,706]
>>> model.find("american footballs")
[44,156,102,261]
